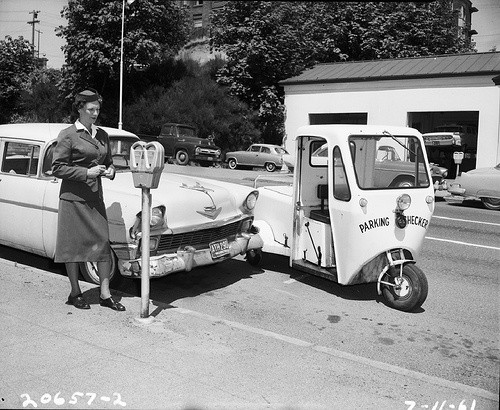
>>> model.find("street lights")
[117,0,137,156]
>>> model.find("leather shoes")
[68,293,90,308]
[99,296,125,311]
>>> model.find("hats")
[71,87,100,104]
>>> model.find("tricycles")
[245,124,435,313]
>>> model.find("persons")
[51,87,125,310]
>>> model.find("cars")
[422,124,477,146]
[373,145,448,188]
[443,163,500,210]
[282,143,354,173]
[225,143,289,172]
[0,122,259,291]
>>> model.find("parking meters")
[129,140,165,318]
[453,151,464,177]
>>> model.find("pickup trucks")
[136,122,221,167]
